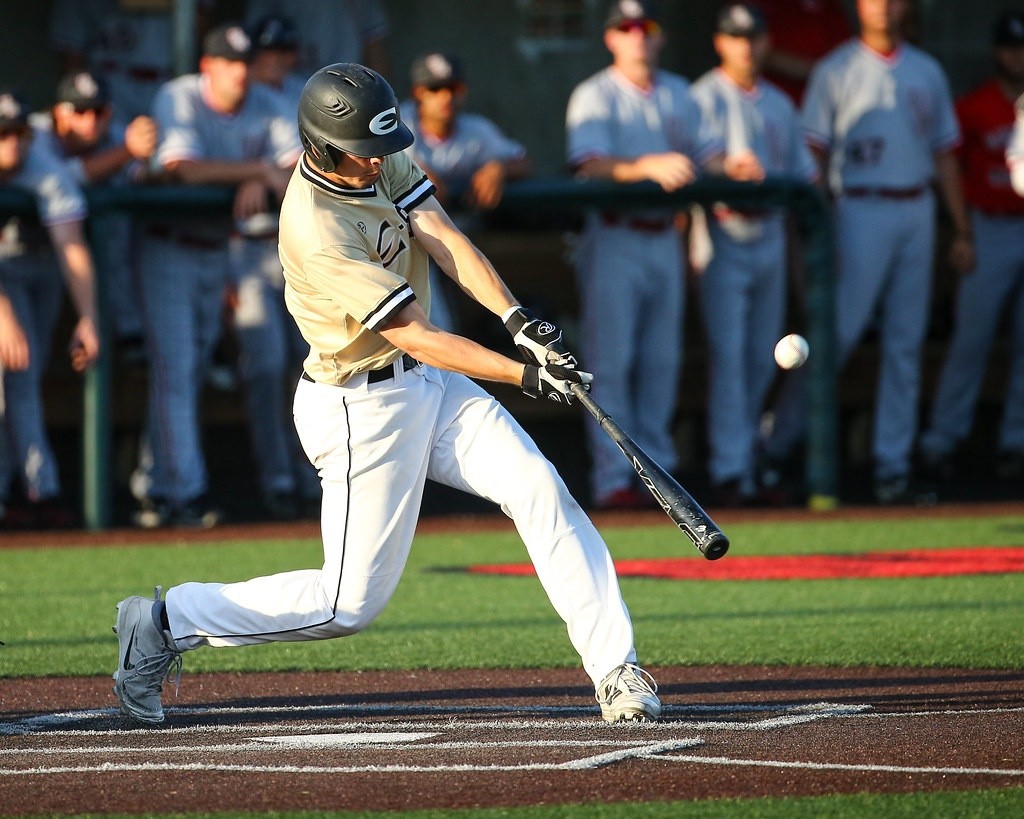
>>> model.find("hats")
[603,0,655,30]
[56,69,115,110]
[411,51,465,87]
[200,25,254,64]
[717,4,768,37]
[1,94,27,138]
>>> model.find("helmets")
[297,61,415,174]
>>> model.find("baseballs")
[773,333,809,370]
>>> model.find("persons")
[0,0,1024,528]
[112,61,661,724]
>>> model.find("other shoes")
[130,495,224,531]
[603,453,1024,511]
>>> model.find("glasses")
[425,81,456,92]
[73,103,105,117]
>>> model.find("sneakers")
[112,584,184,725]
[594,662,661,722]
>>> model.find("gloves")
[521,364,594,406]
[500,304,580,371]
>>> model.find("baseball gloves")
[550,358,729,561]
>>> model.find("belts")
[600,212,689,231]
[302,353,425,384]
[848,186,925,198]
